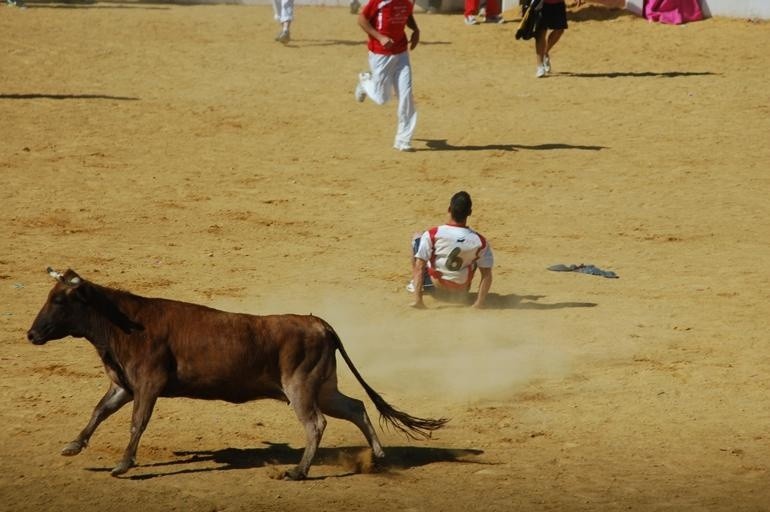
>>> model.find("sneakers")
[465,16,504,24]
[275,30,290,43]
[407,281,425,292]
[394,142,414,152]
[536,54,551,77]
[356,81,366,103]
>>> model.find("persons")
[464,0,506,26]
[406,190,494,309]
[355,0,420,150]
[526,1,569,78]
[270,1,295,44]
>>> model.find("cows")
[24,265,453,481]
